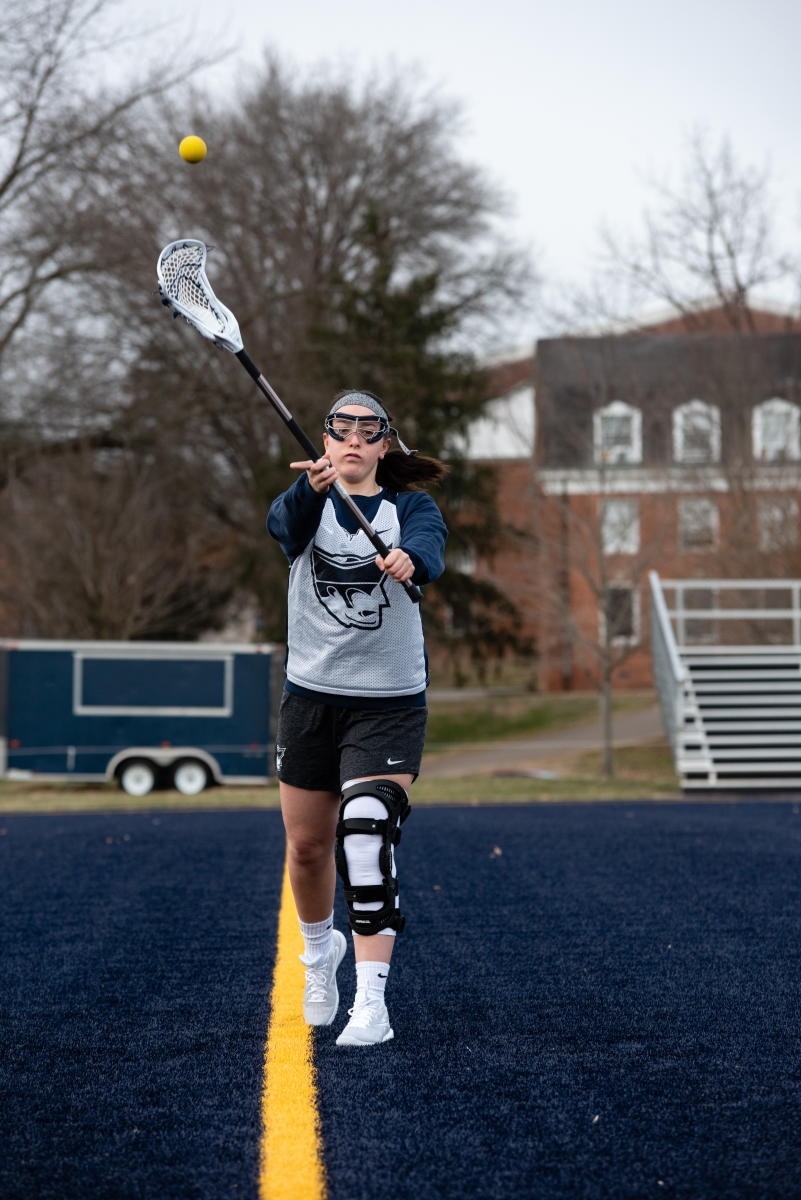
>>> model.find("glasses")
[324,413,389,444]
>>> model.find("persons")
[261,387,449,1049]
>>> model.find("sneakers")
[299,929,347,1027]
[335,999,394,1046]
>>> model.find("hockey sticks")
[151,236,427,608]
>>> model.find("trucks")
[0,637,281,797]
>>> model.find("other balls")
[178,132,206,164]
